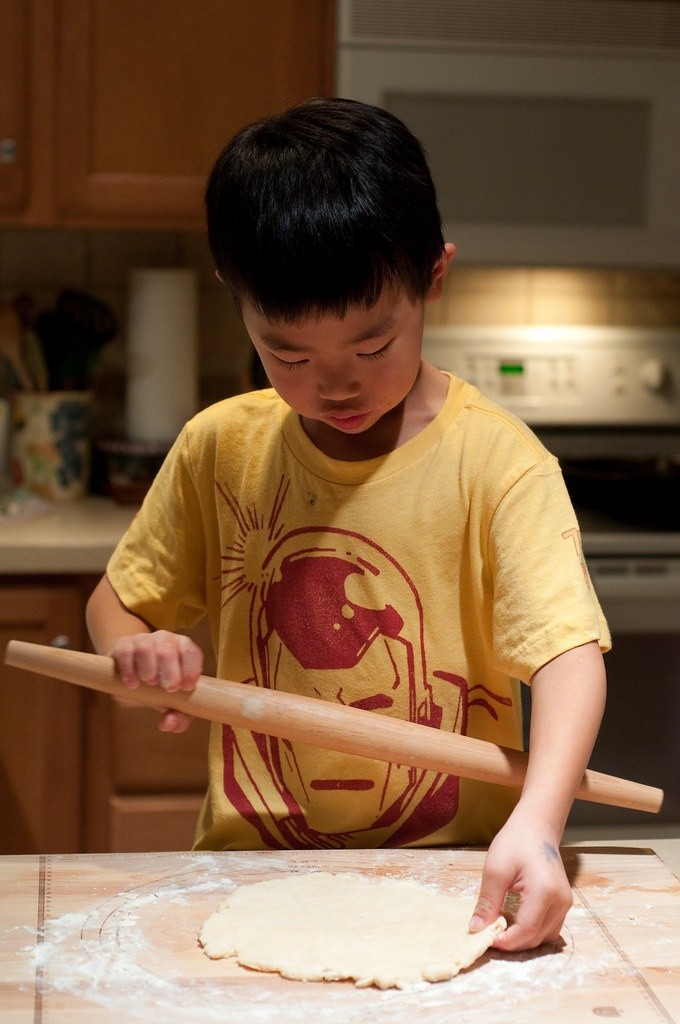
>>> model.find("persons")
[84,92,609,963]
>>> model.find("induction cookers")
[419,322,680,635]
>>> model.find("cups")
[6,392,93,502]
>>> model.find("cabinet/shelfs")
[0,572,218,856]
[0,0,347,233]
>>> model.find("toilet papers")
[127,267,197,442]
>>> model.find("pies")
[194,872,510,991]
